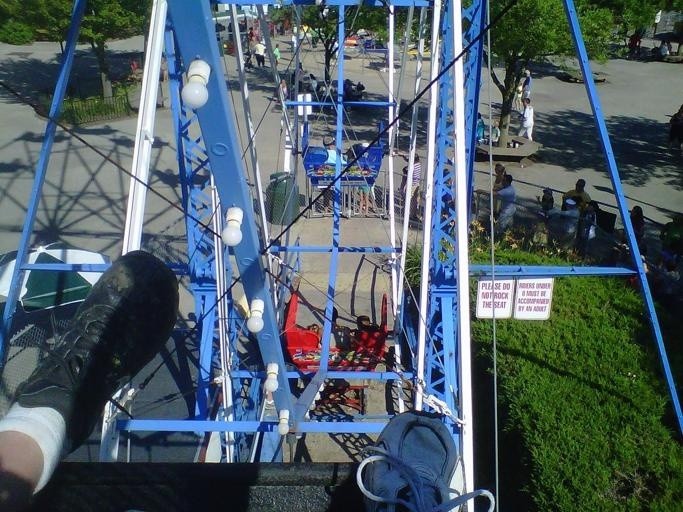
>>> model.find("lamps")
[262,360,279,394]
[221,205,244,251]
[243,298,265,333]
[176,59,212,112]
[276,409,289,436]
[293,383,325,442]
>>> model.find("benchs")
[469,134,542,162]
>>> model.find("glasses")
[329,140,335,145]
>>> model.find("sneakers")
[355,410,495,512]
[9,251,180,455]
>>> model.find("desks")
[287,67,368,112]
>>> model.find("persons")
[349,316,370,352]
[514,83,523,110]
[626,30,641,60]
[252,35,365,110]
[509,98,535,147]
[319,135,452,222]
[655,39,669,60]
[668,105,683,157]
[519,70,531,102]
[492,120,500,146]
[476,163,683,315]
[317,307,352,351]
[666,40,672,53]
[477,113,486,141]
[0,250,178,512]
